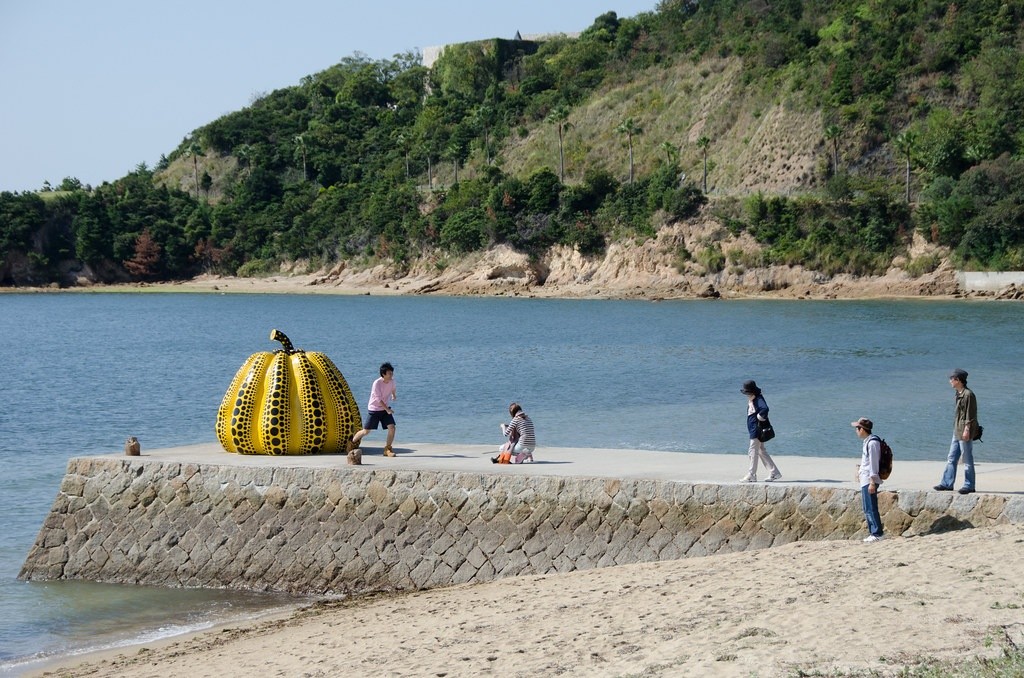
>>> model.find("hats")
[850,417,873,430]
[740,380,761,395]
[947,368,968,380]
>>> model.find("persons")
[345,362,397,457]
[851,417,884,542]
[740,380,782,482]
[932,367,977,494]
[490,403,536,464]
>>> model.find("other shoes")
[958,487,975,494]
[933,484,953,491]
[383,445,396,457]
[527,456,533,462]
[347,441,357,454]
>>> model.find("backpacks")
[866,435,893,479]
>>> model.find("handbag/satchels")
[755,423,775,442]
[973,426,983,441]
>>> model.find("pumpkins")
[216,329,362,456]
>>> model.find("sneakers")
[864,534,883,542]
[764,472,782,482]
[739,473,757,482]
